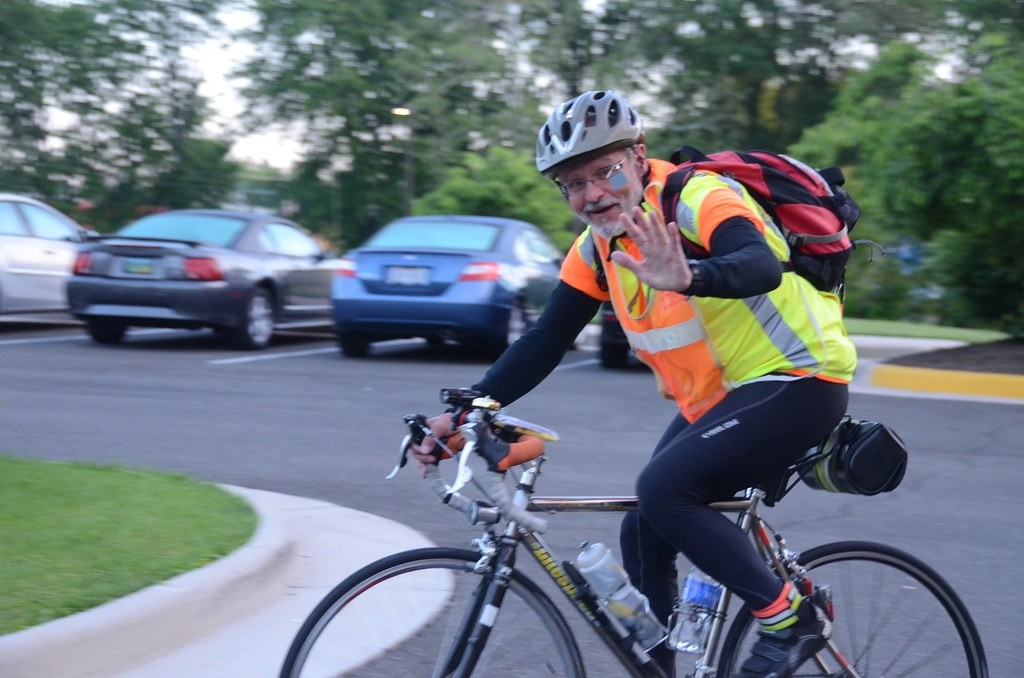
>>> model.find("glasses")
[558,144,638,196]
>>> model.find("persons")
[411,89,859,678]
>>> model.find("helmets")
[535,90,641,178]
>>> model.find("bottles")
[575,540,664,649]
[668,566,724,655]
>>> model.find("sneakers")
[735,581,833,678]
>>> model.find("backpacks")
[594,146,862,294]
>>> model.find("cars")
[328,213,565,366]
[66,207,340,350]
[0,191,100,326]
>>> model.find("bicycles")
[278,378,990,677]
